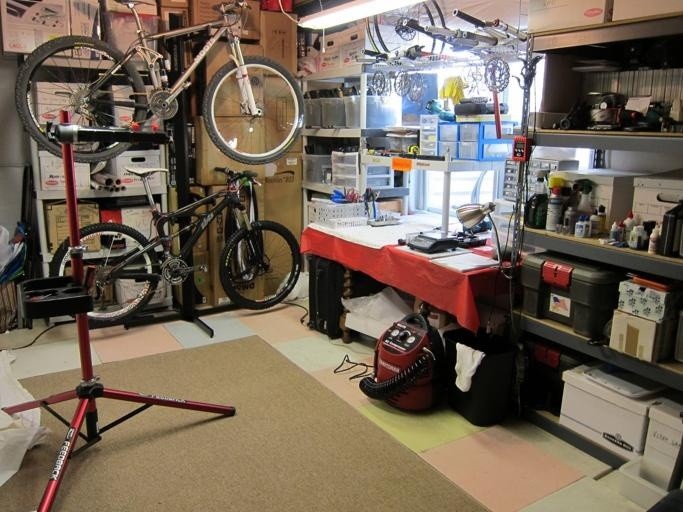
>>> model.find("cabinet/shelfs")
[505,15,682,471]
[22,55,175,326]
[365,152,505,235]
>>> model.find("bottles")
[545,185,663,255]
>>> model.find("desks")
[298,211,528,343]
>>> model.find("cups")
[593,149,602,168]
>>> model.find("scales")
[407,230,459,253]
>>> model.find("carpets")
[0,333,494,512]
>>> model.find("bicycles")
[46,167,304,330]
[12,0,308,167]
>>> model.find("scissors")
[347,188,359,203]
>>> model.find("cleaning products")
[524,169,597,231]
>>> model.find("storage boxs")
[103,83,159,129]
[1,0,159,62]
[161,2,302,308]
[609,309,679,364]
[43,201,102,254]
[520,251,622,339]
[631,169,682,251]
[64,265,114,308]
[560,365,666,456]
[115,262,175,308]
[296,3,402,222]
[100,205,164,248]
[618,279,681,324]
[644,400,683,471]
[34,81,92,129]
[564,170,653,232]
[103,141,160,192]
[38,140,92,190]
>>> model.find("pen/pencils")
[364,188,380,202]
[330,186,362,203]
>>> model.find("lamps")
[455,201,504,272]
